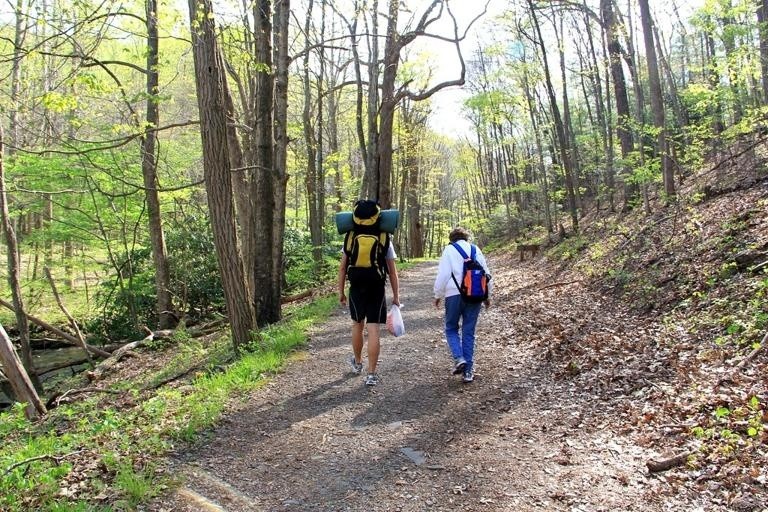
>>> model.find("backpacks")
[447,241,489,302]
[345,229,390,288]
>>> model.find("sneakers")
[463,372,474,382]
[366,375,377,386]
[350,355,364,374]
[451,359,467,376]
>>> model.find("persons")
[336,201,400,387]
[432,226,493,383]
